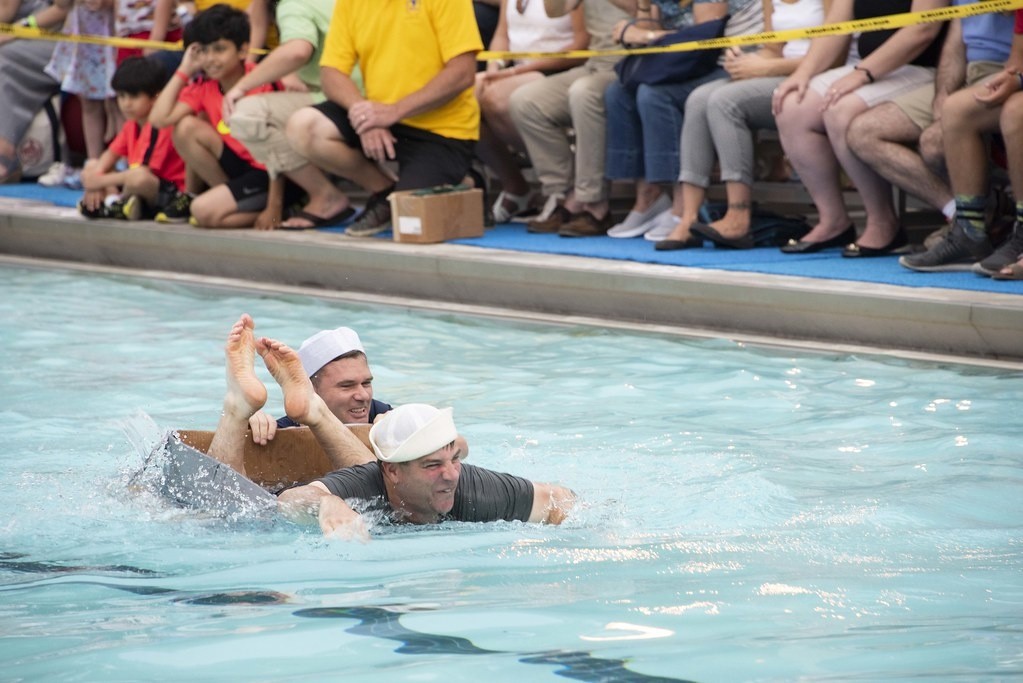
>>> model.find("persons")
[245,324,397,448]
[203,312,578,537]
[0,0,1023,280]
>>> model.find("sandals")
[992,253,1023,280]
[0,156,22,185]
[492,190,535,222]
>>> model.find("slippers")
[274,207,356,229]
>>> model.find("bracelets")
[508,66,516,76]
[854,65,874,83]
[495,57,506,68]
[647,31,654,40]
[1016,72,1023,91]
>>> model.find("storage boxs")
[388,188,485,244]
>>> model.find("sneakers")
[109,195,142,220]
[557,211,609,237]
[899,221,995,271]
[36,162,70,186]
[345,191,391,236]
[155,192,192,223]
[527,205,576,232]
[974,222,1023,274]
[645,215,683,240]
[65,168,81,189]
[77,199,109,219]
[606,192,673,238]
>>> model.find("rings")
[773,88,778,95]
[361,115,367,121]
[832,88,837,93]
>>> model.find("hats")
[295,327,365,379]
[367,403,459,464]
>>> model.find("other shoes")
[689,223,754,249]
[923,224,953,249]
[841,225,912,256]
[655,235,702,250]
[780,223,856,253]
